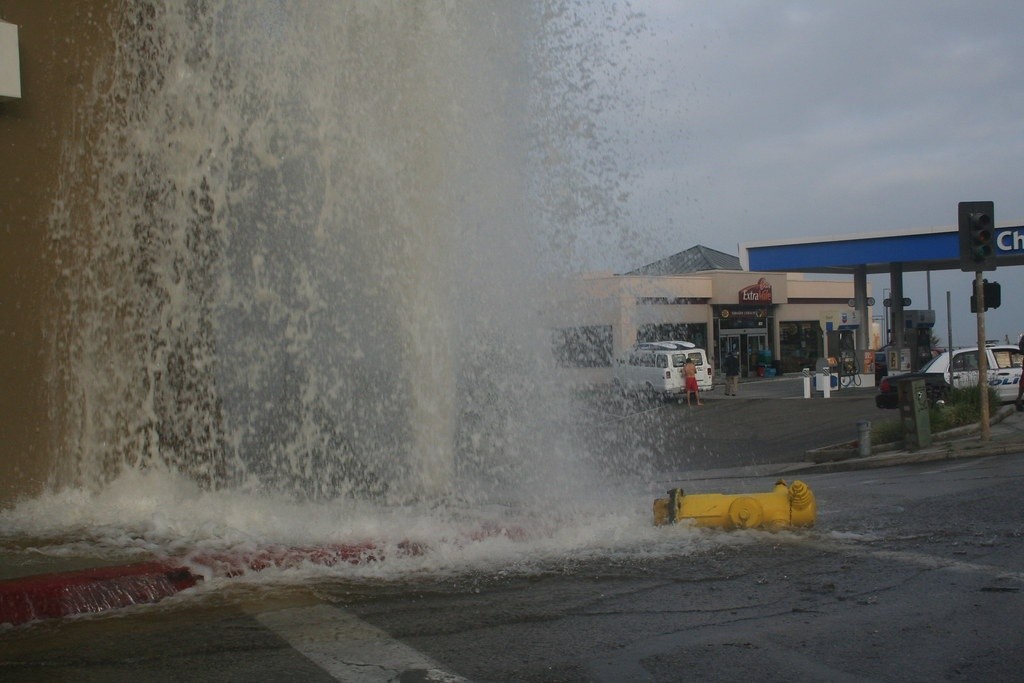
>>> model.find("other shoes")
[725,393,730,396]
[732,394,736,396]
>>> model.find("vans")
[611,344,714,403]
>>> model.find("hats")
[727,352,733,355]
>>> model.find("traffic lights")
[958,202,999,272]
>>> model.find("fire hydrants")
[652,476,818,535]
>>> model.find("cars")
[874,342,1023,412]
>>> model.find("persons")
[683,358,704,408]
[723,352,739,396]
